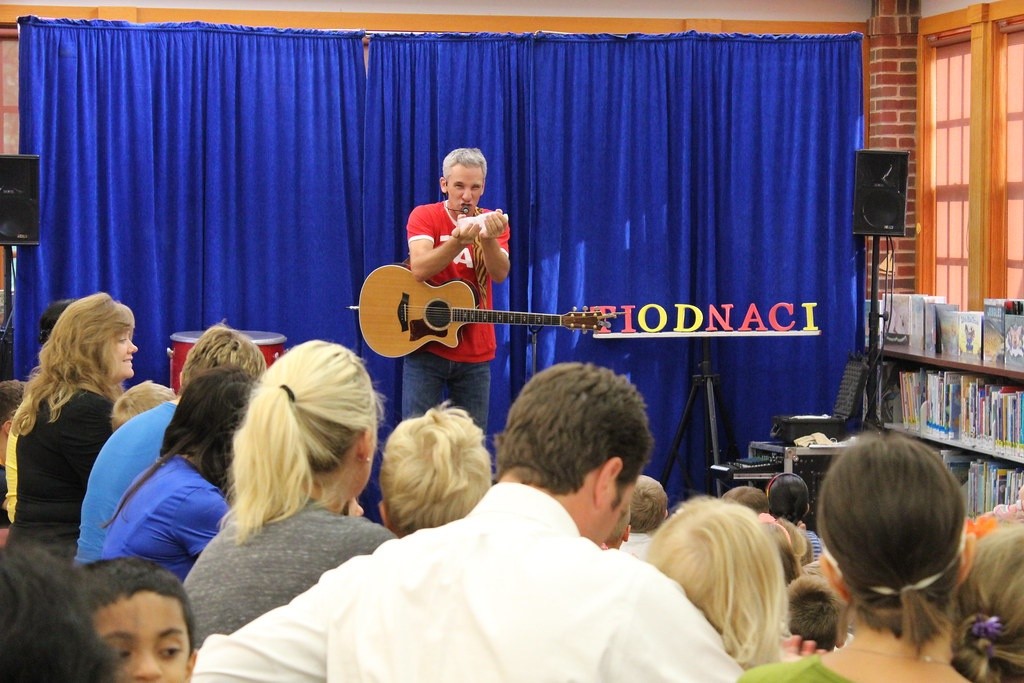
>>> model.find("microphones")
[459,206,469,214]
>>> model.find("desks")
[592,329,821,497]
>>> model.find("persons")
[950,526,1024,683]
[402,148,510,448]
[738,432,976,683]
[0,291,846,683]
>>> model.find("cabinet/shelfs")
[864,344,1024,467]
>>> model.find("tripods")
[659,339,743,499]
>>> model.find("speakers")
[0,155,40,245]
[853,150,911,236]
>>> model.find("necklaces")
[848,643,953,667]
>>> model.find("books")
[866,293,1024,372]
[936,449,1024,523]
[899,367,1024,460]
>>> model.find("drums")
[167,327,289,398]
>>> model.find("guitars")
[346,262,605,358]
